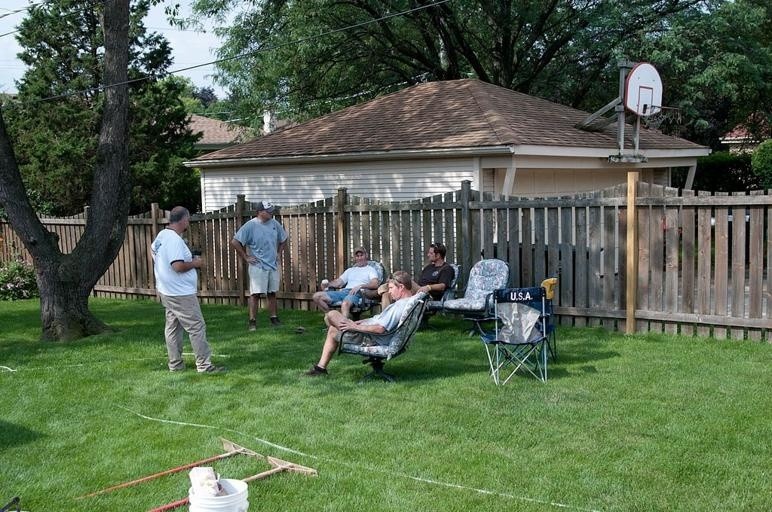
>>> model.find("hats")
[256,200,276,213]
[354,247,367,255]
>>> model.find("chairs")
[421,263,459,333]
[491,277,559,377]
[488,286,553,387]
[339,291,431,383]
[443,257,511,341]
[324,260,385,321]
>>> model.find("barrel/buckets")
[187,478,250,512]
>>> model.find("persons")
[312,247,382,321]
[230,200,290,331]
[149,206,225,373]
[304,269,428,376]
[378,242,455,312]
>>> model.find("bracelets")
[426,284,432,290]
[359,285,363,289]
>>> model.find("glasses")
[389,274,401,283]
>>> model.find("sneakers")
[204,366,226,373]
[306,365,328,375]
[249,320,257,332]
[270,317,287,328]
